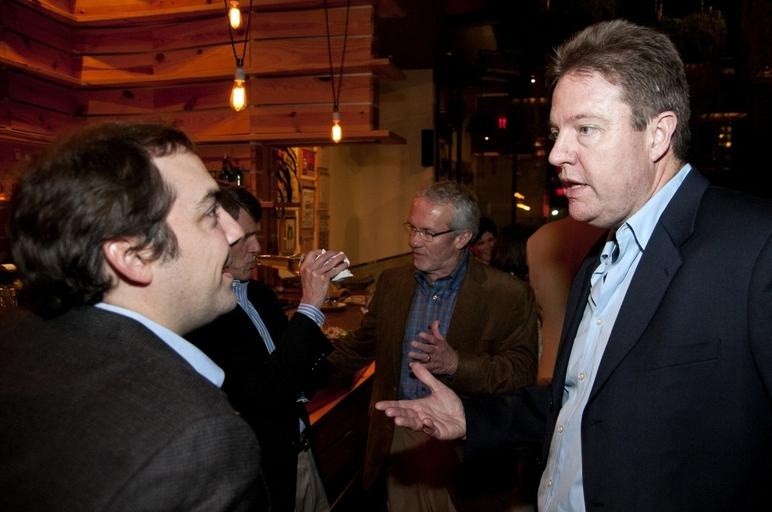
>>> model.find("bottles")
[253,253,344,276]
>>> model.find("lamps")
[226,1,251,112]
[228,0,242,28]
[323,0,350,144]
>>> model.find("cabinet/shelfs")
[300,145,315,256]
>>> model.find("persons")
[466,217,528,285]
[181,185,348,511]
[323,180,541,512]
[0,118,278,509]
[526,212,610,389]
[374,20,772,512]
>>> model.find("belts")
[298,436,309,451]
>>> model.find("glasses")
[403,223,456,242]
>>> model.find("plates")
[321,302,347,309]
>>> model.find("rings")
[427,354,432,362]
